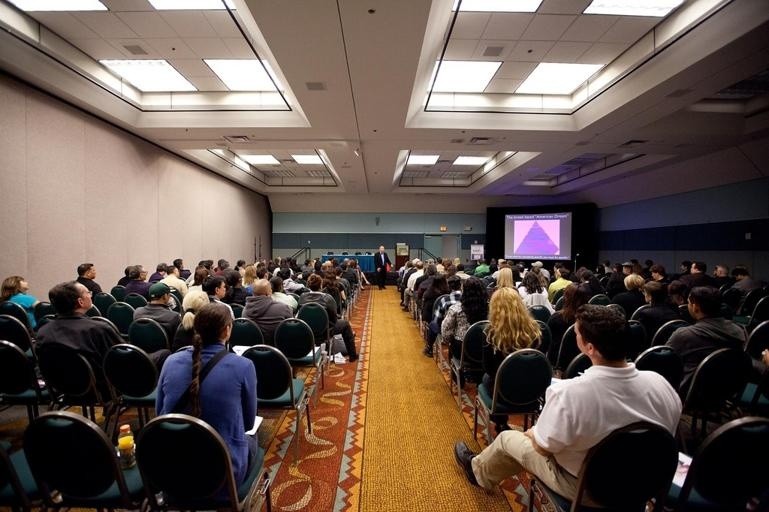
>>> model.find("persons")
[1,276,39,334]
[35,281,127,418]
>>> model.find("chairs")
[0,264,362,511]
[401,265,768,511]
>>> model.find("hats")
[531,260,544,268]
[148,282,171,299]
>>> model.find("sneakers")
[424,347,433,358]
[378,286,386,290]
[349,354,359,362]
[400,302,409,312]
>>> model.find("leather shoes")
[454,441,479,486]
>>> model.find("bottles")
[116,423,136,471]
